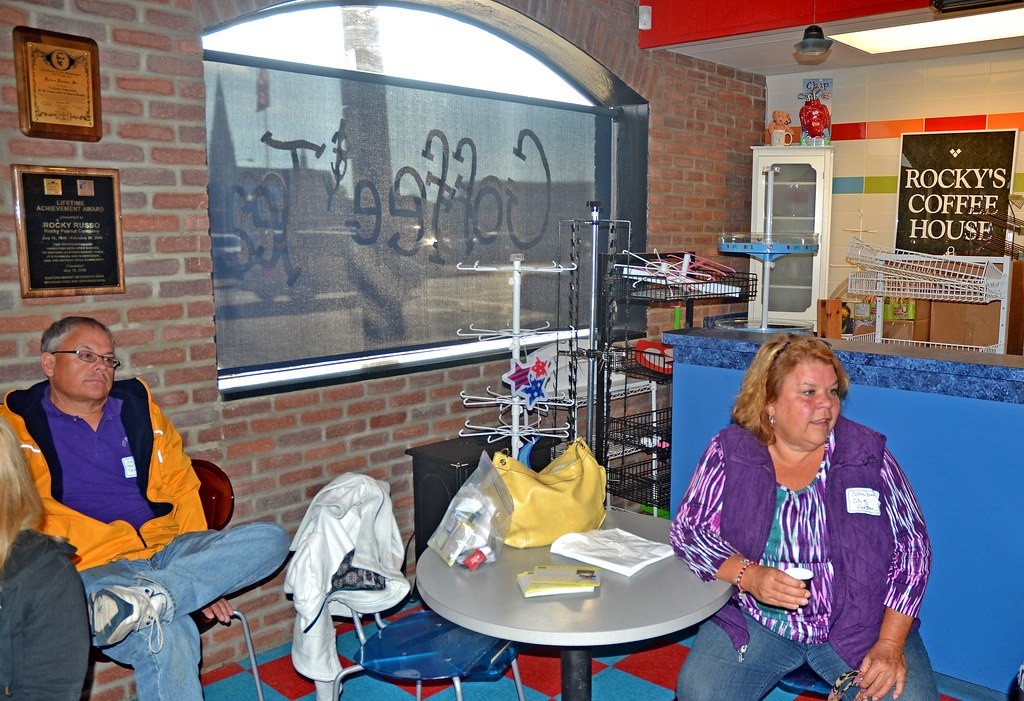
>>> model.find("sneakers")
[88,585,166,654]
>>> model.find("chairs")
[189,459,265,701]
[332,601,527,701]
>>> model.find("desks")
[405,429,563,570]
[415,510,732,701]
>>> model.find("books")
[516,564,602,598]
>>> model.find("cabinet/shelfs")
[747,145,834,330]
[595,251,757,510]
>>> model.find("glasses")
[51,350,120,368]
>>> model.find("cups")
[770,130,793,146]
[784,568,814,608]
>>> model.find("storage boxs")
[847,294,1000,346]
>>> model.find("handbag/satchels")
[483,436,607,550]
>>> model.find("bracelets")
[734,558,757,599]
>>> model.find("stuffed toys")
[767,110,794,136]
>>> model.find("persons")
[664,328,943,700]
[0,316,290,701]
[0,412,89,700]
